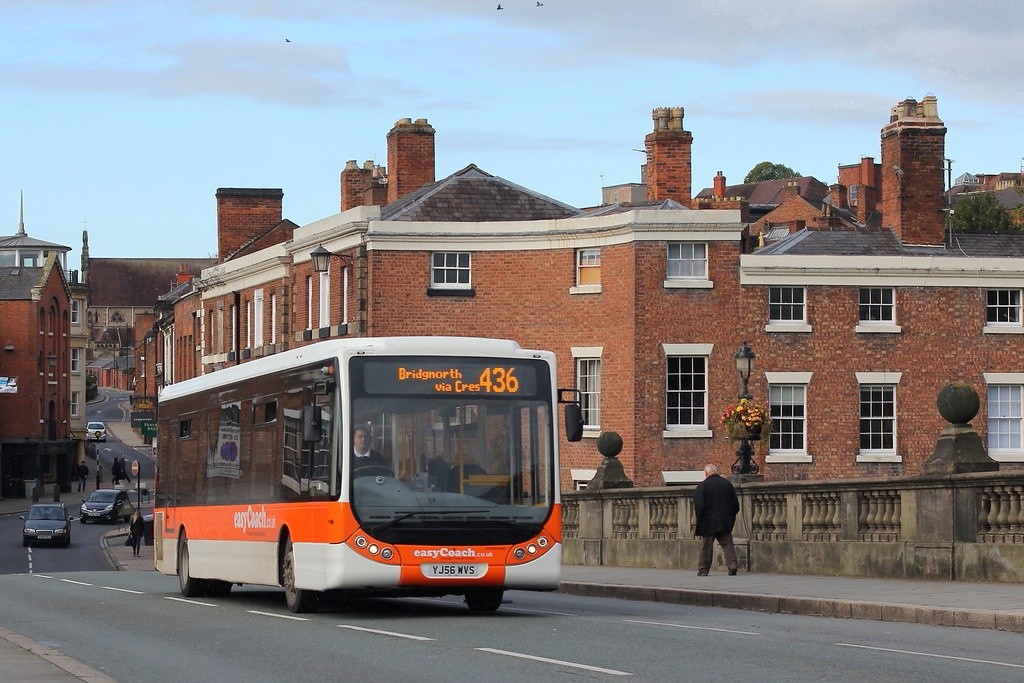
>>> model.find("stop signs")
[131,462,139,476]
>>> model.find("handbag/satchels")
[124,533,133,546]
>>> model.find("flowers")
[719,398,773,446]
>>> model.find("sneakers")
[696,570,709,575]
[727,568,737,575]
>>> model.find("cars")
[87,421,108,440]
[20,504,73,548]
[80,488,129,526]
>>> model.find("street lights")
[96,431,100,490]
[150,332,585,613]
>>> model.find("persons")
[76,460,89,492]
[352,427,389,479]
[129,510,145,557]
[110,457,121,485]
[118,452,132,483]
[693,463,740,576]
